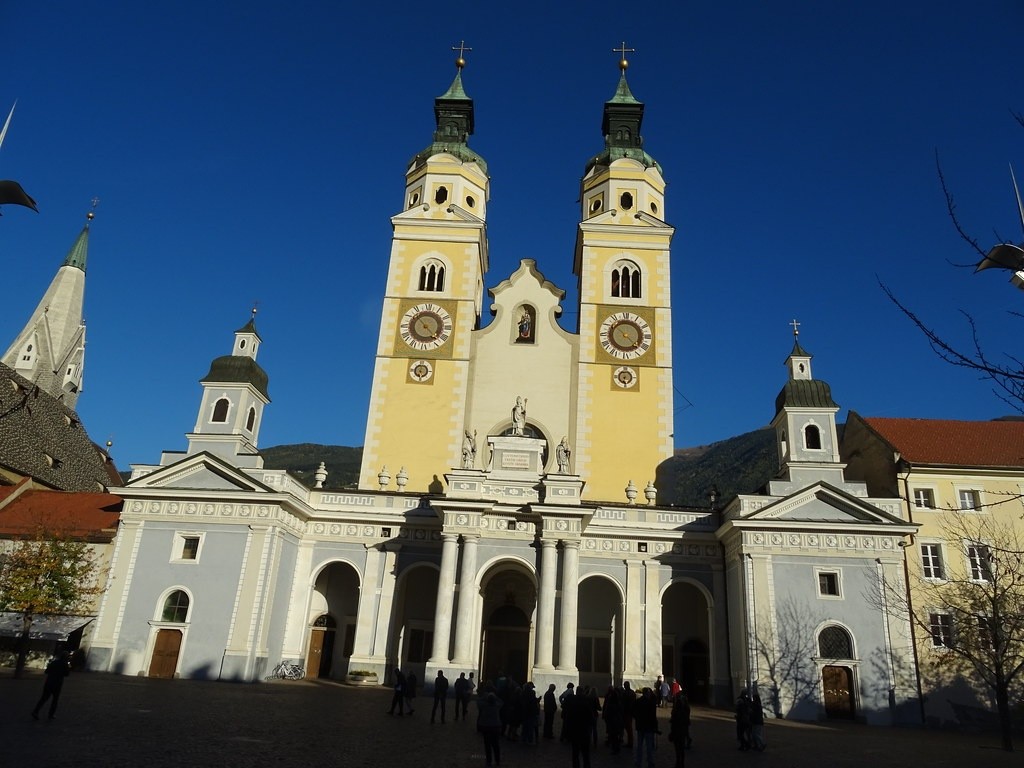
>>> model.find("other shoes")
[526,740,541,746]
[738,743,751,752]
[396,713,403,716]
[386,711,393,715]
[543,734,556,739]
[611,748,620,754]
[406,710,414,716]
[753,743,766,752]
[455,715,466,720]
[442,720,447,724]
[430,718,434,723]
[622,744,632,748]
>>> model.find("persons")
[462,430,476,468]
[390,668,764,768]
[512,397,526,435]
[556,437,570,473]
[32,651,70,722]
[522,310,530,336]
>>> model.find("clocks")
[405,359,436,386]
[609,365,640,392]
[393,299,458,359]
[595,305,657,367]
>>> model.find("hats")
[408,669,416,675]
[567,683,574,687]
[393,668,398,672]
